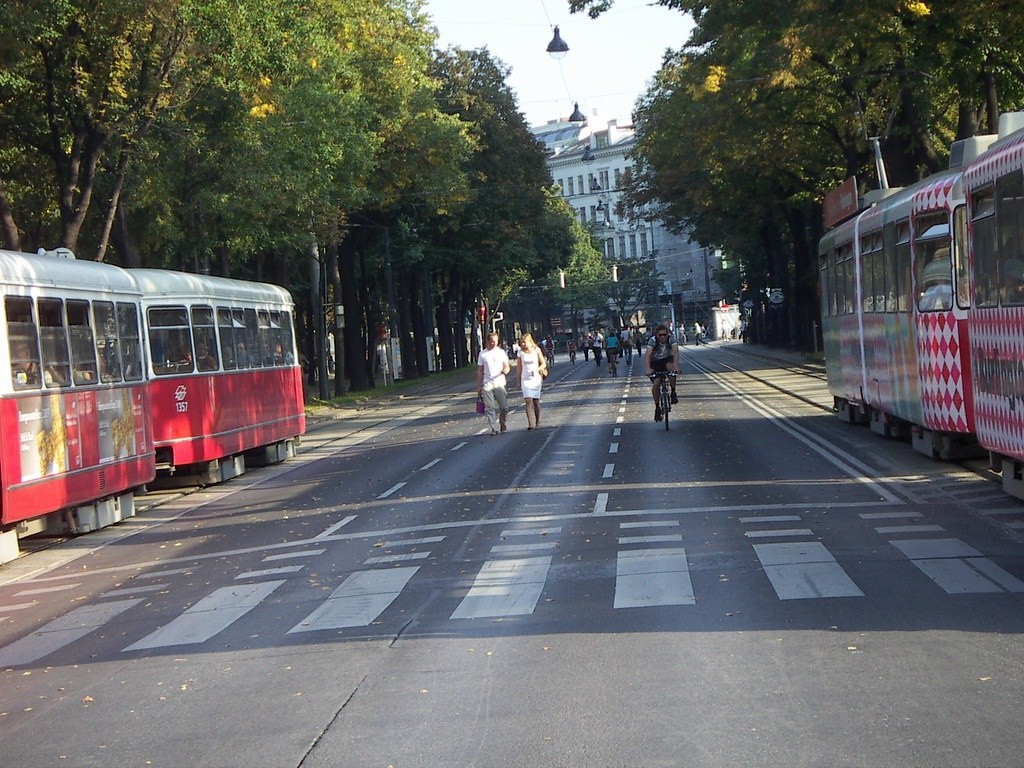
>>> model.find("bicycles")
[645,368,682,432]
[609,353,619,376]
[546,347,556,367]
[568,346,578,366]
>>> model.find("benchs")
[154,355,216,374]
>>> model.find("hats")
[921,247,951,283]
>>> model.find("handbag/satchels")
[476,395,485,413]
[539,366,549,378]
[593,341,600,347]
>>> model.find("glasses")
[658,333,667,337]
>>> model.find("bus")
[1,246,307,544]
[818,112,1024,500]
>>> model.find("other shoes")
[671,393,678,404]
[491,431,498,436]
[608,370,612,374]
[535,421,541,431]
[499,425,507,432]
[616,361,619,364]
[527,427,533,432]
[654,406,662,421]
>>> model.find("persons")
[517,333,546,431]
[10,340,142,384]
[498,325,652,368]
[664,323,712,346]
[645,325,680,422]
[436,342,440,361]
[976,234,1024,305]
[739,317,747,344]
[605,332,620,374]
[299,350,310,374]
[148,315,294,373]
[477,333,510,436]
